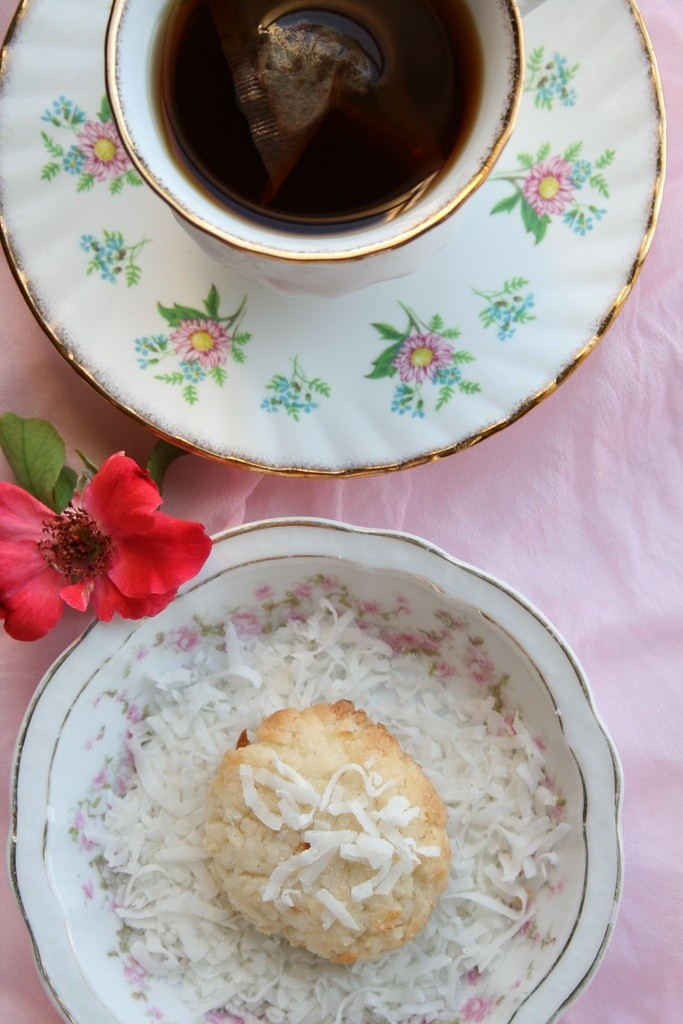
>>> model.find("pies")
[207,698,449,964]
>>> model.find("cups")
[103,0,551,265]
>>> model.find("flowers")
[0,410,215,645]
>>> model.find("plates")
[8,514,624,1023]
[0,1,667,479]
